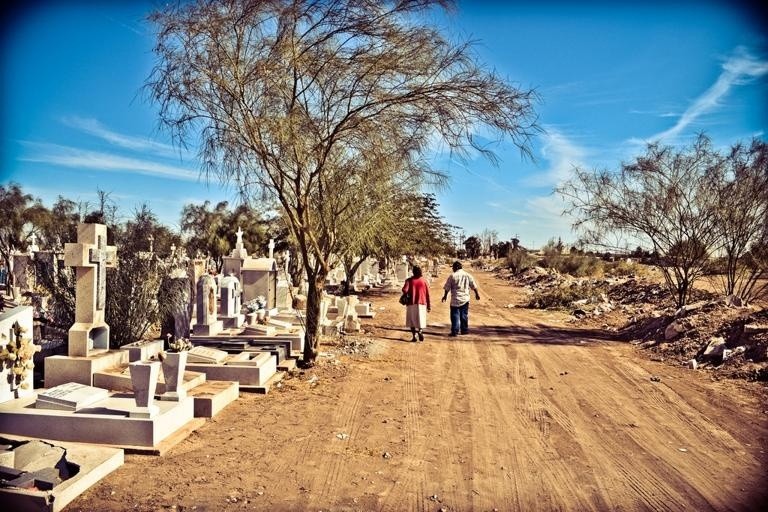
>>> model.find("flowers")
[0,320,42,396]
[149,332,194,361]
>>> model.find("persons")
[399,266,431,342]
[442,261,480,336]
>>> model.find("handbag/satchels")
[398,278,411,306]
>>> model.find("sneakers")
[410,336,418,342]
[447,332,458,337]
[418,332,424,341]
[460,330,469,335]
